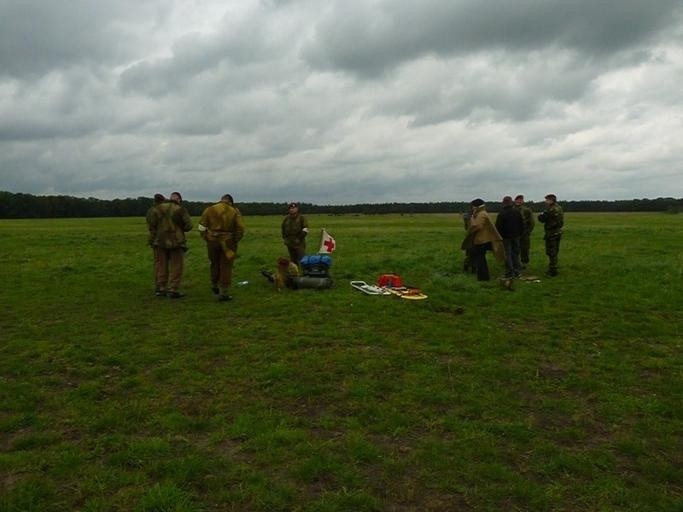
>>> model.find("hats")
[155,192,299,210]
[468,195,556,221]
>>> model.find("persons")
[467,198,504,281]
[260,256,299,287]
[458,202,478,273]
[197,192,246,301]
[494,195,522,277]
[280,203,307,265]
[537,193,564,277]
[149,191,193,299]
[514,194,533,270]
[144,193,171,293]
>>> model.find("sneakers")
[503,260,558,280]
[154,284,186,298]
[210,285,233,302]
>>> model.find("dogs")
[278,255,298,278]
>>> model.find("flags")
[317,230,337,255]
